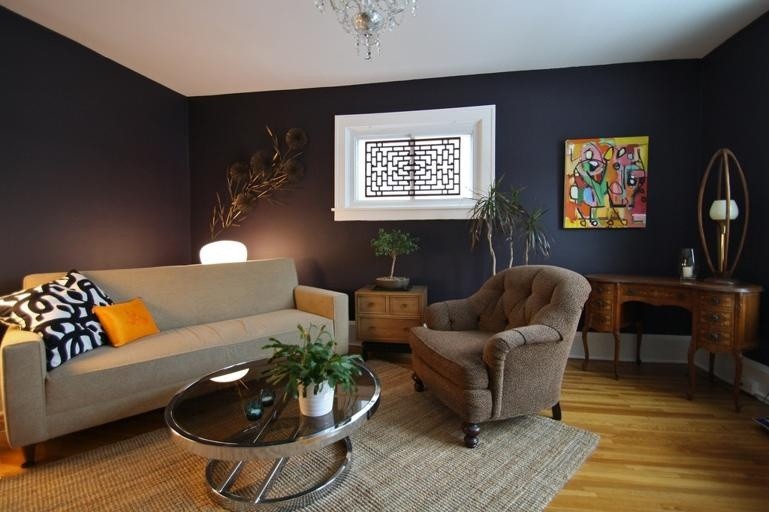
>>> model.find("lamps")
[209,368,250,384]
[696,148,750,286]
[199,240,247,264]
[315,0,417,62]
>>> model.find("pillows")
[92,297,160,348]
[0,269,115,372]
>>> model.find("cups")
[246,398,264,421]
[260,387,276,406]
[680,248,696,280]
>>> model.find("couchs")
[0,257,350,468]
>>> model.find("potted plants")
[370,227,421,291]
[263,322,365,418]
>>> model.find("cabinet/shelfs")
[355,285,427,361]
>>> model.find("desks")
[583,273,763,413]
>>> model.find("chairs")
[409,264,592,448]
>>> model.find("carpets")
[1,360,601,512]
